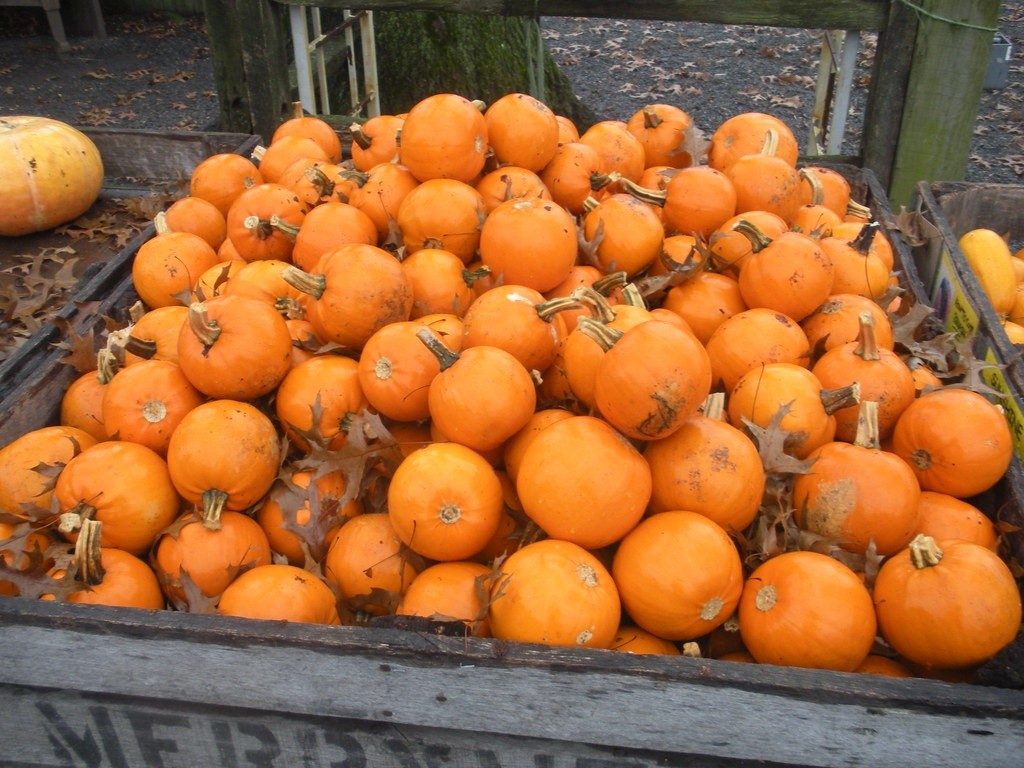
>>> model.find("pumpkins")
[0,93,1024,679]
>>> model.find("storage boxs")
[0,125,1024,768]
